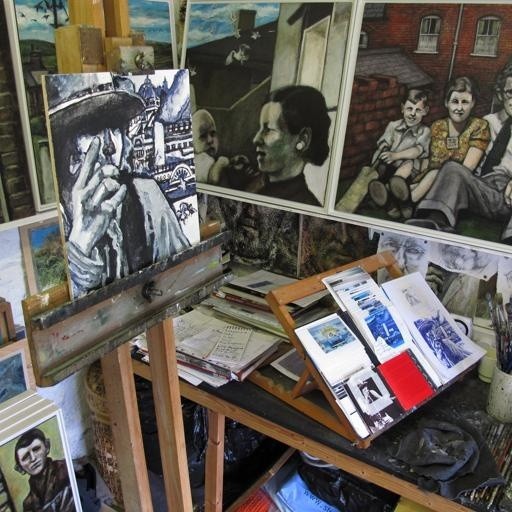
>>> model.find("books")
[134,269,334,390]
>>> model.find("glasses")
[503,88,512,99]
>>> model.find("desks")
[131,347,512,512]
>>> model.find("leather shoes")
[390,174,416,220]
[368,179,401,221]
[403,215,455,236]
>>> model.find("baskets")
[83,361,179,511]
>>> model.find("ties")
[481,116,512,177]
[115,172,149,273]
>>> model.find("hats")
[45,74,144,139]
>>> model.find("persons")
[369,87,432,189]
[190,106,251,185]
[404,60,511,245]
[43,74,191,294]
[204,85,336,278]
[370,73,492,219]
[13,428,75,512]
